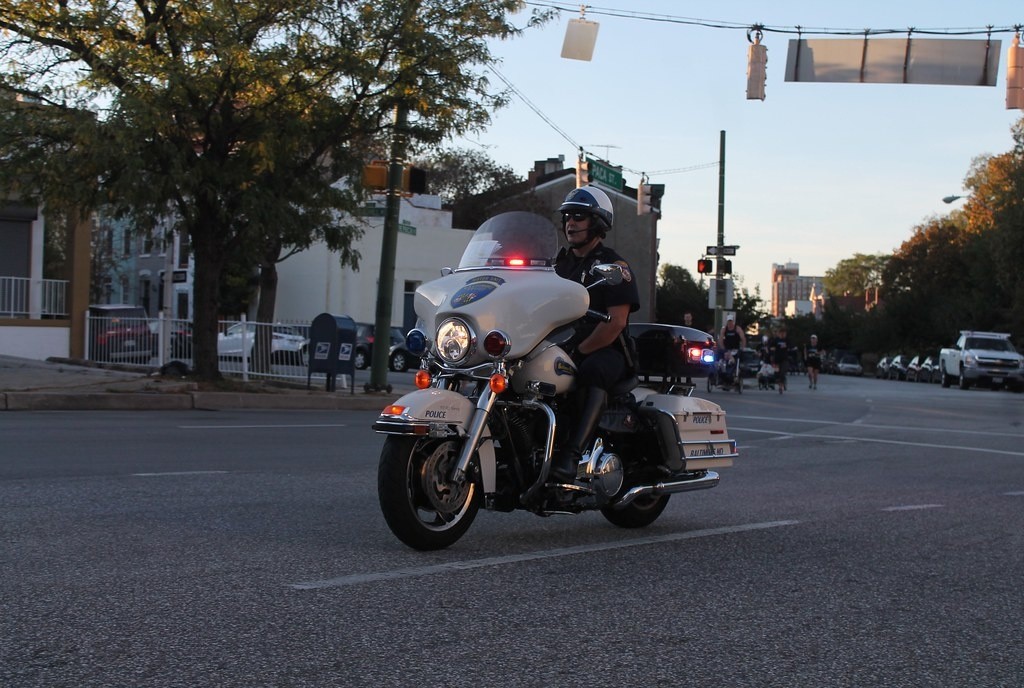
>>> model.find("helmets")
[557,187,614,230]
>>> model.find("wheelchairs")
[705,348,743,393]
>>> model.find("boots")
[548,388,608,485]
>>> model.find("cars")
[874,356,895,380]
[627,322,717,377]
[905,355,929,383]
[388,336,425,372]
[837,354,863,377]
[739,347,762,376]
[145,320,192,356]
[216,322,309,367]
[351,321,406,369]
[920,355,960,384]
[888,355,914,381]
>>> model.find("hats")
[811,335,818,339]
[727,315,734,321]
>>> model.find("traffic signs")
[706,246,736,257]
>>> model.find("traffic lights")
[405,163,428,196]
[697,259,712,273]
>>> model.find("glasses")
[563,211,593,222]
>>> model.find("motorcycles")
[367,210,742,554]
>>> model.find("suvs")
[54,302,155,365]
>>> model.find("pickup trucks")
[938,330,1024,391]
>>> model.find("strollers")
[755,349,787,391]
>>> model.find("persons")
[681,313,825,394]
[548,187,635,486]
